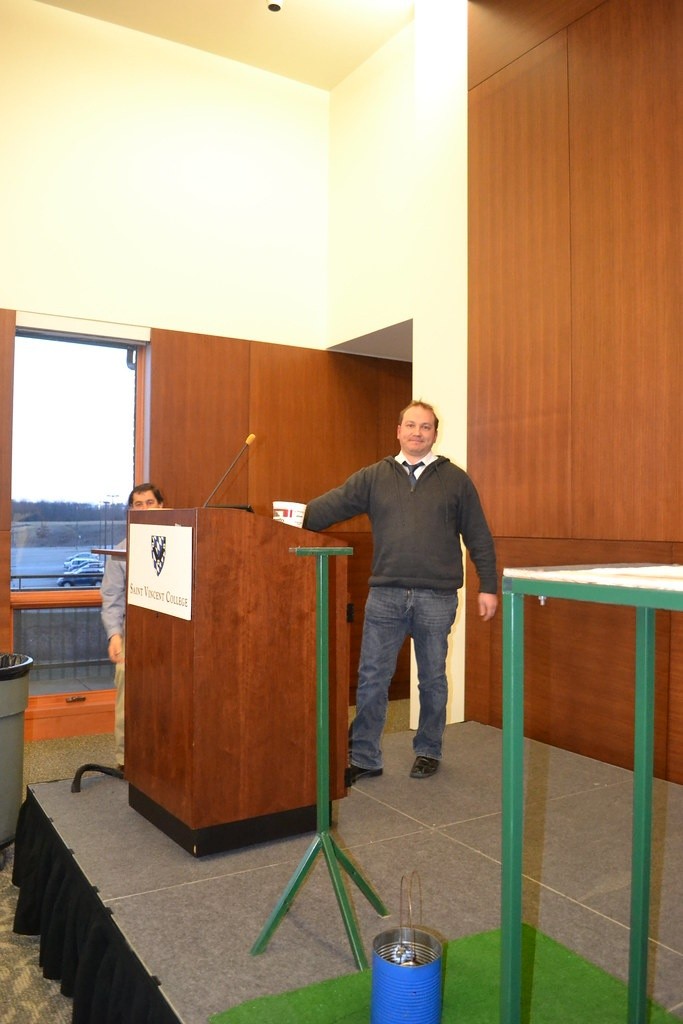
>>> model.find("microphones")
[203,434,256,508]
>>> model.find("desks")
[499,561,683,1024]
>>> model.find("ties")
[402,461,425,485]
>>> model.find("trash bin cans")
[0,653,34,852]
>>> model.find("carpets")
[203,923,683,1024]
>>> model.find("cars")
[57,551,105,588]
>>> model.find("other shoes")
[410,755,439,778]
[345,764,383,787]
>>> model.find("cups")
[272,500,308,529]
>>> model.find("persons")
[99,483,163,772]
[302,399,498,784]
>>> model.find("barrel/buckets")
[370,875,442,1024]
